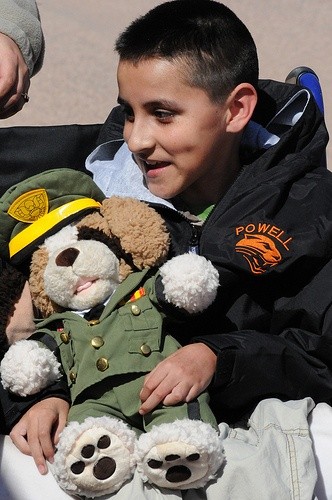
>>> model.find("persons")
[0,0,332,500]
[0,0,43,119]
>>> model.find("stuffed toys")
[0,168,221,500]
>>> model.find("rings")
[21,92,29,102]
